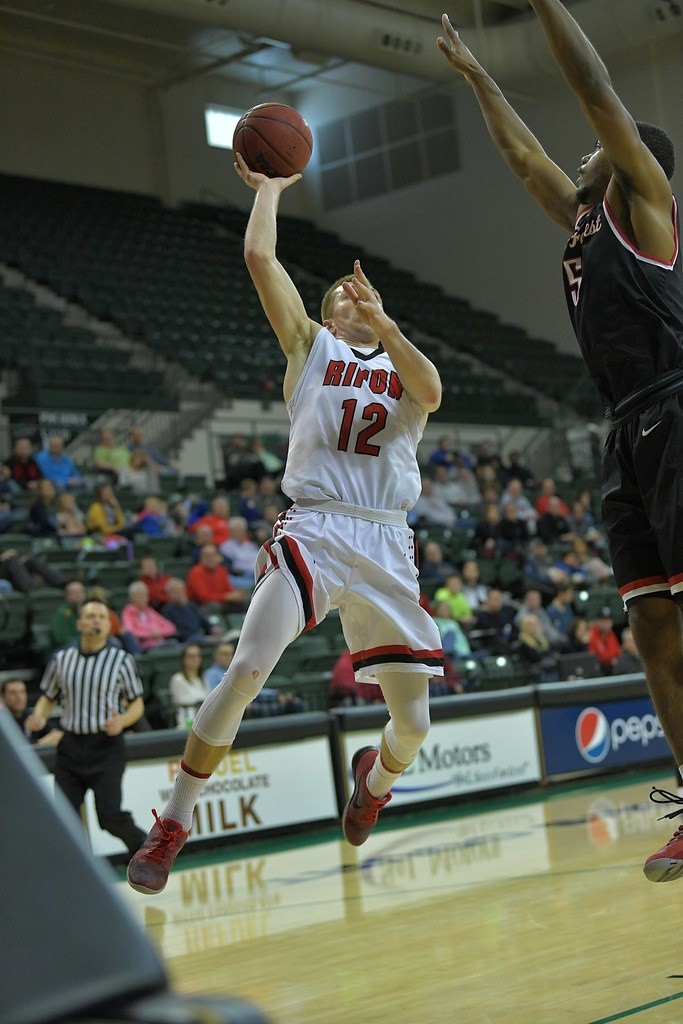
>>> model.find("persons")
[28,594,148,860]
[3,679,64,748]
[206,639,292,703]
[168,643,213,730]
[0,424,287,648]
[436,1,683,886]
[121,153,447,899]
[414,431,648,700]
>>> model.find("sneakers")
[342,745,393,847]
[126,807,191,894]
[640,786,682,883]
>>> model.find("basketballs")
[232,102,313,178]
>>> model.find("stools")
[0,169,645,750]
